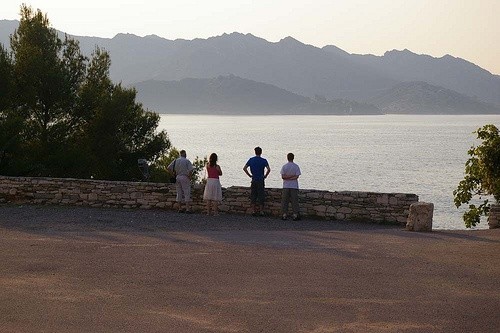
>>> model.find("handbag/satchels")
[171,175,176,183]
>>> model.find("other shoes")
[186,210,193,214]
[179,210,185,213]
[293,217,300,221]
[206,214,210,216]
[214,214,220,217]
[282,216,287,220]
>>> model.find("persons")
[202,153,223,217]
[280,153,302,220]
[168,150,194,214]
[243,147,271,217]
